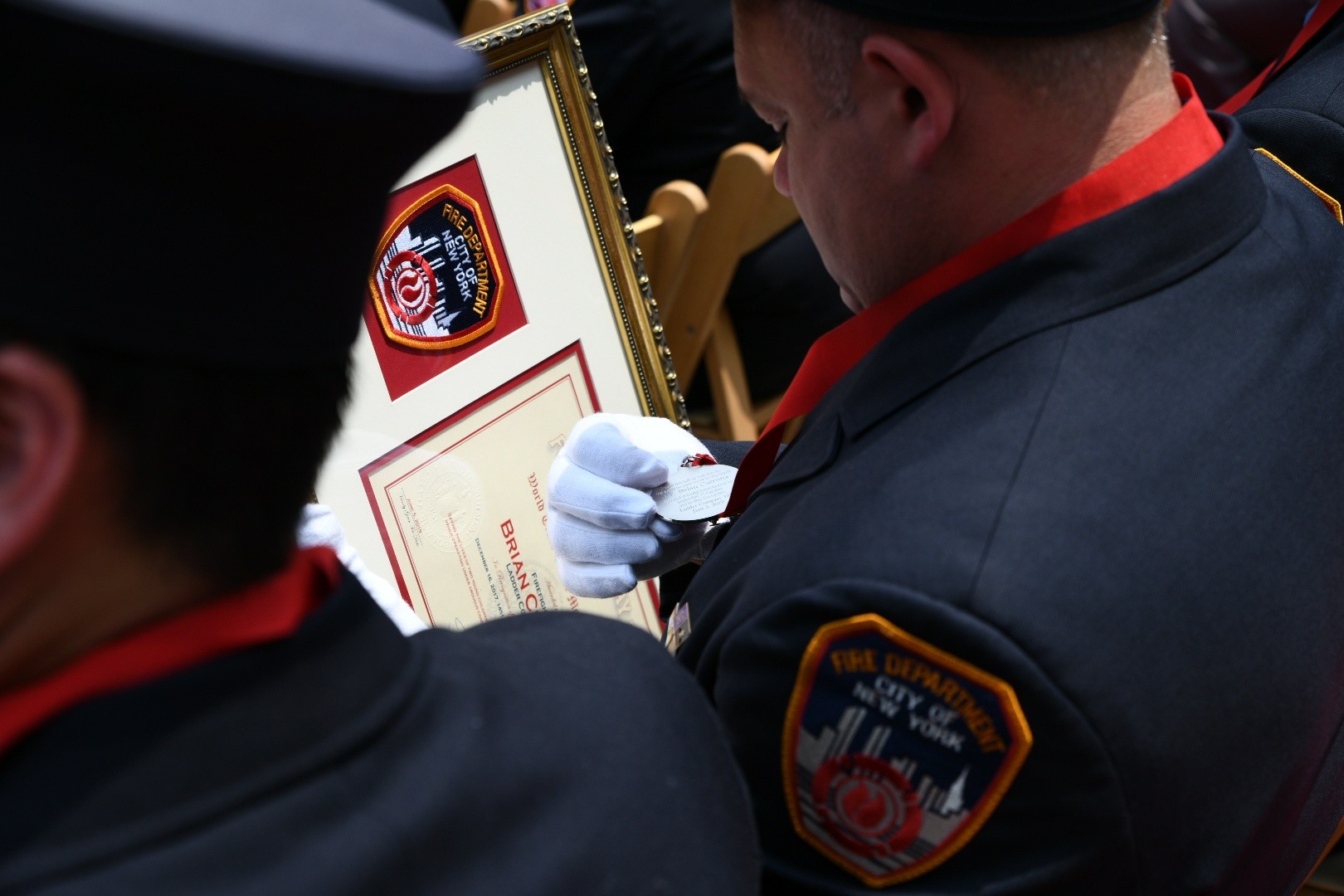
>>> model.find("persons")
[0,0,764,896]
[542,0,1344,896]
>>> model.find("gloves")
[545,412,717,599]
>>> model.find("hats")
[0,0,483,268]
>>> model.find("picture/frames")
[279,2,695,677]
